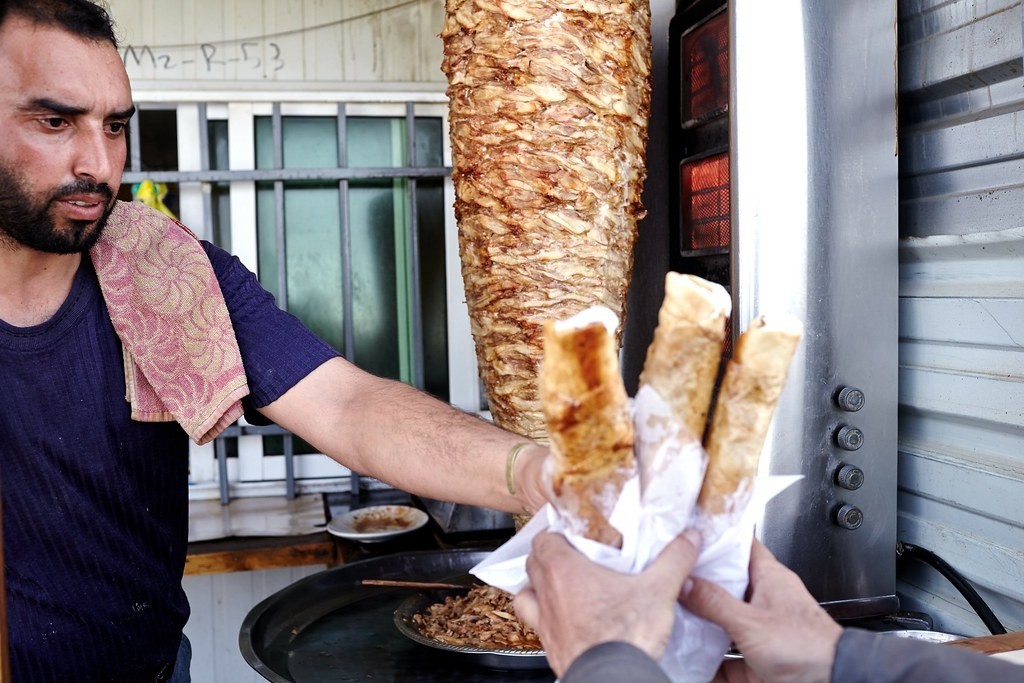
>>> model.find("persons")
[511,524,1024,683]
[0,0,635,683]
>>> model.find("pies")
[540,273,798,551]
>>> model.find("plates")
[325,504,428,543]
[392,591,552,669]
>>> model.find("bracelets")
[505,441,537,514]
